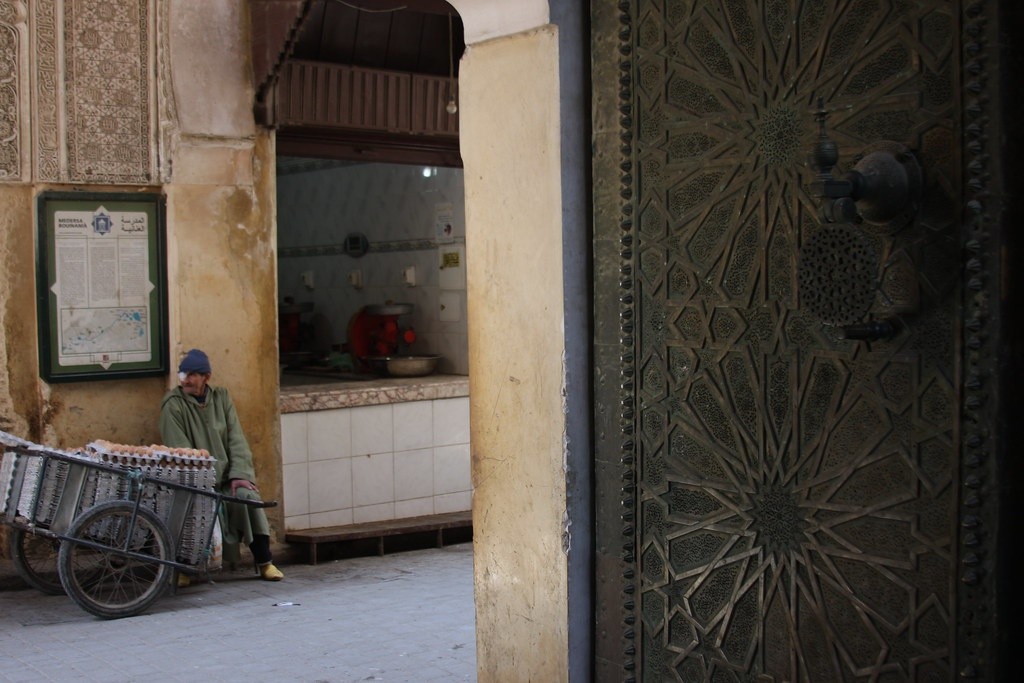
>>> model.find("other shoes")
[170,574,190,587]
[261,563,284,580]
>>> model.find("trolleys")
[0,431,278,620]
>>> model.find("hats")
[178,348,211,373]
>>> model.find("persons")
[159,349,283,586]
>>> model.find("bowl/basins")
[375,353,443,378]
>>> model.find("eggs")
[66,439,211,470]
